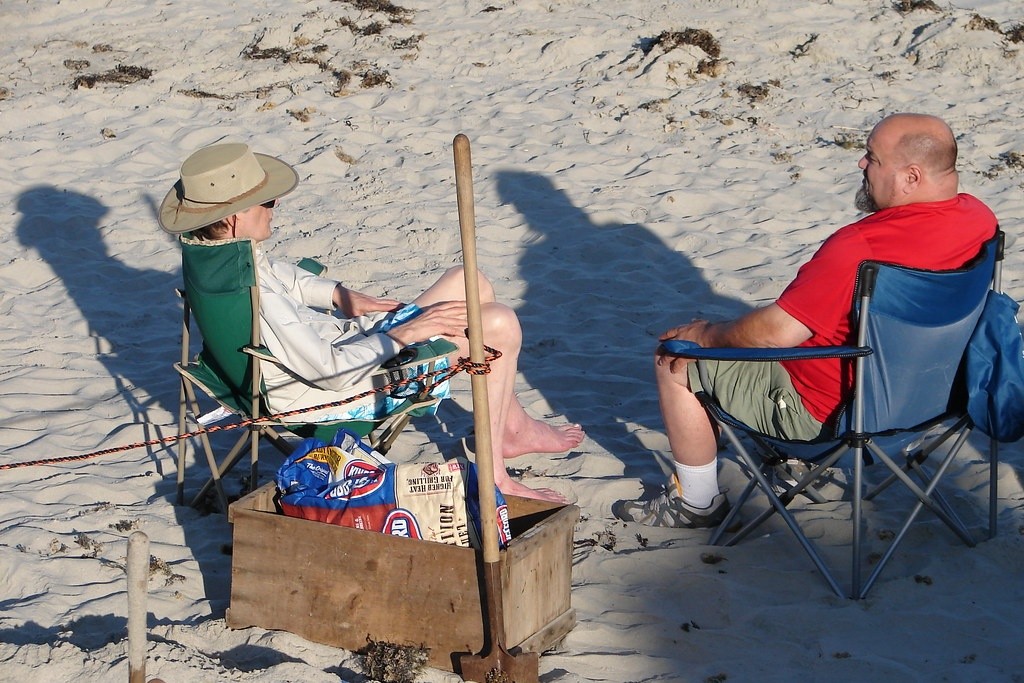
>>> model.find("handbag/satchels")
[277,428,512,550]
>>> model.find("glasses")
[261,200,276,208]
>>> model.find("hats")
[158,143,300,234]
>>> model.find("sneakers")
[776,462,834,492]
[611,473,742,531]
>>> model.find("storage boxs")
[225,474,580,673]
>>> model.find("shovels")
[452,133,539,683]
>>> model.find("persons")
[179,143,585,505]
[611,113,998,528]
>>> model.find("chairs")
[656,227,1008,602]
[171,233,460,517]
